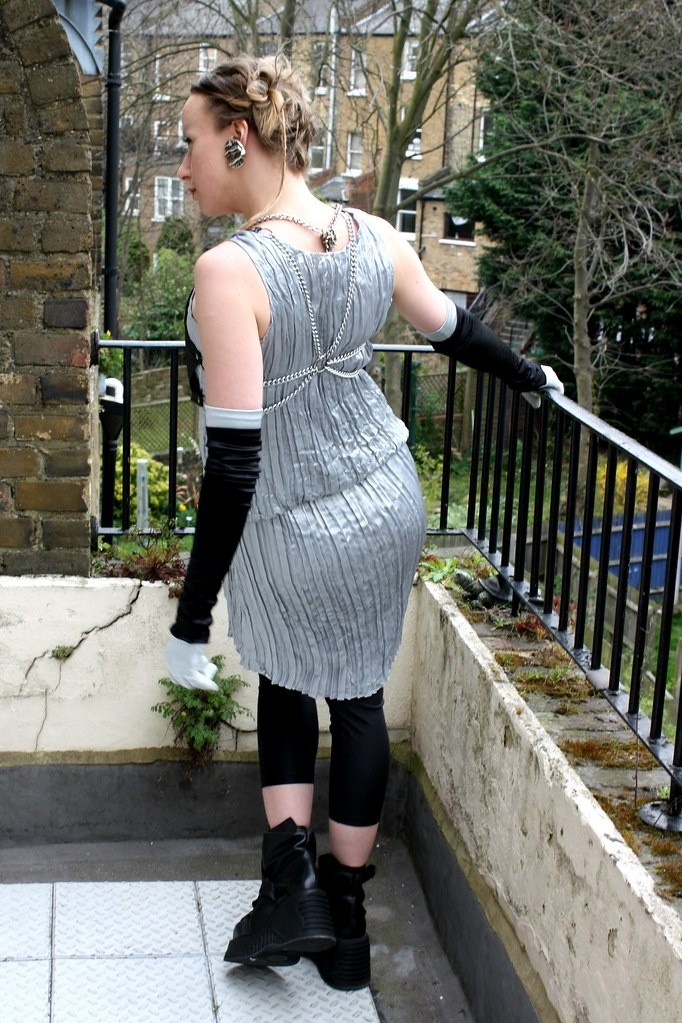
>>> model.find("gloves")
[520,363,564,409]
[162,627,219,693]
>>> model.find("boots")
[222,816,335,969]
[302,853,372,992]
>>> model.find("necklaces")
[245,198,344,251]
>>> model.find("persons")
[163,54,564,992]
